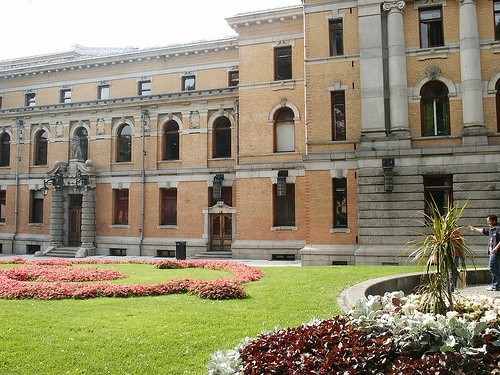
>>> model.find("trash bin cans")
[175,240,187,260]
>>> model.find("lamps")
[277,177,287,197]
[213,180,222,199]
[382,166,395,193]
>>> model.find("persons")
[467,214,500,292]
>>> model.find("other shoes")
[486,287,497,291]
